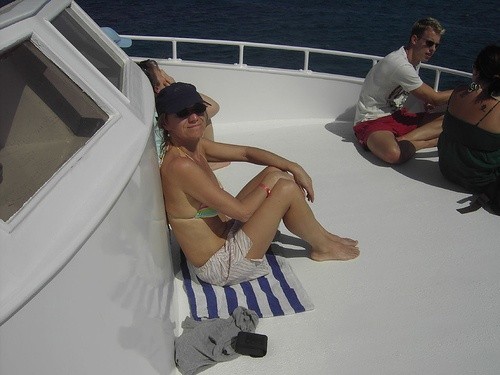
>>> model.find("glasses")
[419,37,439,49]
[175,103,207,118]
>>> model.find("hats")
[100,27,132,48]
[155,82,204,114]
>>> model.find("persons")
[352,15,454,166]
[138,59,231,171]
[437,45,500,216]
[154,83,360,286]
[101,27,132,48]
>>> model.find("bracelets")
[260,182,272,197]
